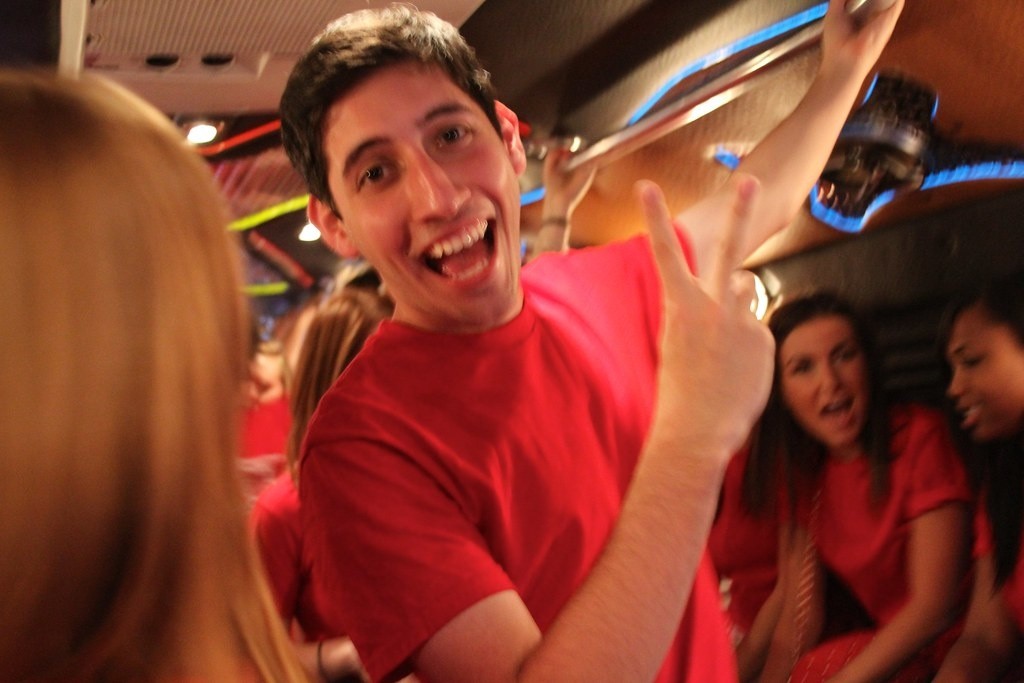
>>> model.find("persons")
[0,0,1024,682]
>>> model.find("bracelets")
[540,216,570,229]
[315,638,329,681]
